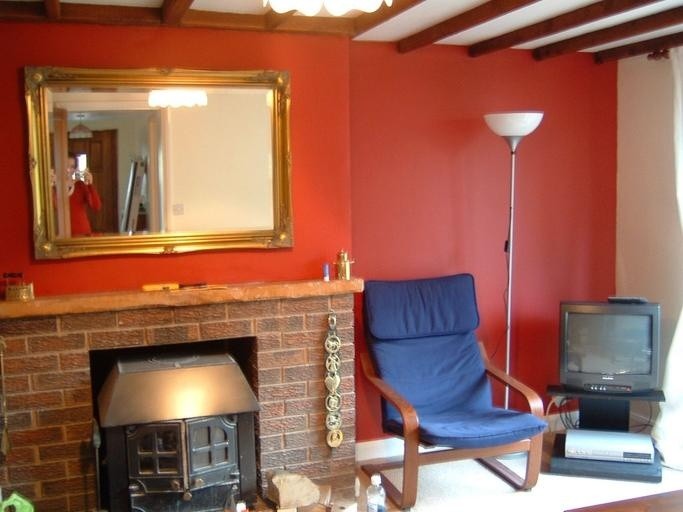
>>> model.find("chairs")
[358,272,548,508]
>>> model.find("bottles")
[366,475,385,512]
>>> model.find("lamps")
[67,118,95,141]
[145,91,208,110]
[261,0,392,18]
[482,110,544,462]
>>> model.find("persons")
[49,153,102,236]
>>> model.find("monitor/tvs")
[557,300,661,393]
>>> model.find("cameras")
[70,172,86,182]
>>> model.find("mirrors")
[21,62,293,266]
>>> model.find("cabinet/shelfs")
[539,386,665,481]
